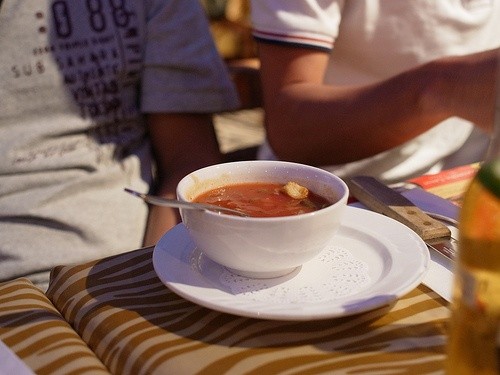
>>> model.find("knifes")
[341,174,461,265]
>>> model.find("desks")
[0,160,488,375]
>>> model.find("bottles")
[446,84,500,375]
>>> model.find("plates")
[151,205,432,320]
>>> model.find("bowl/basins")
[175,159,350,279]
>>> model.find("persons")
[250,1,499,190]
[0,1,240,297]
